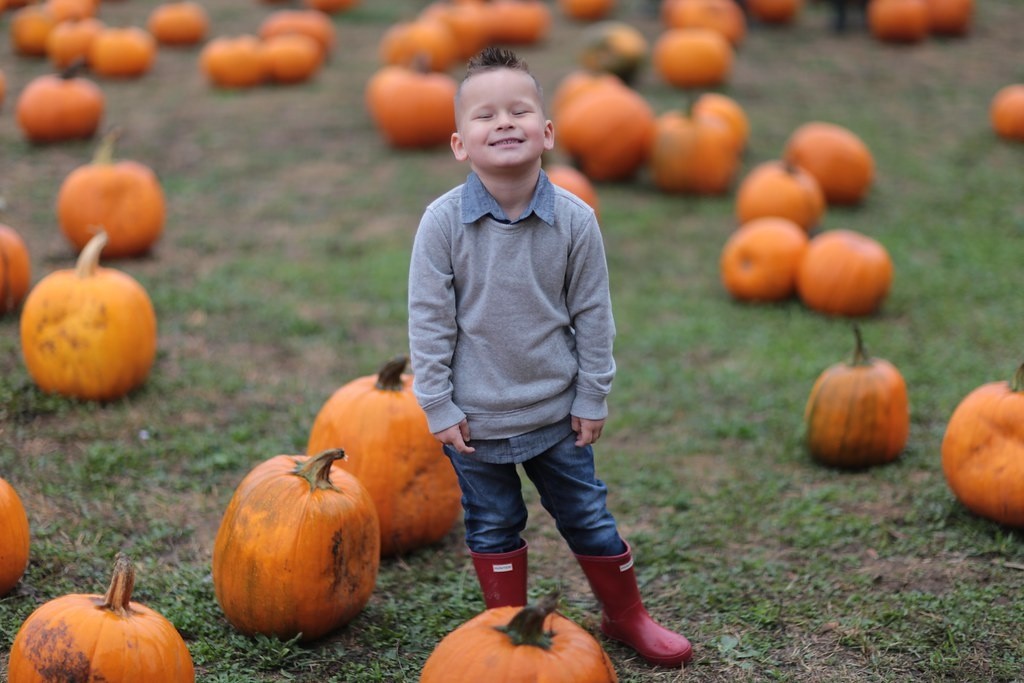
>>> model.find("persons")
[406,46,694,669]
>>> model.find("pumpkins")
[0,0,1024,683]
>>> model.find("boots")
[572,534,692,668]
[468,536,528,609]
